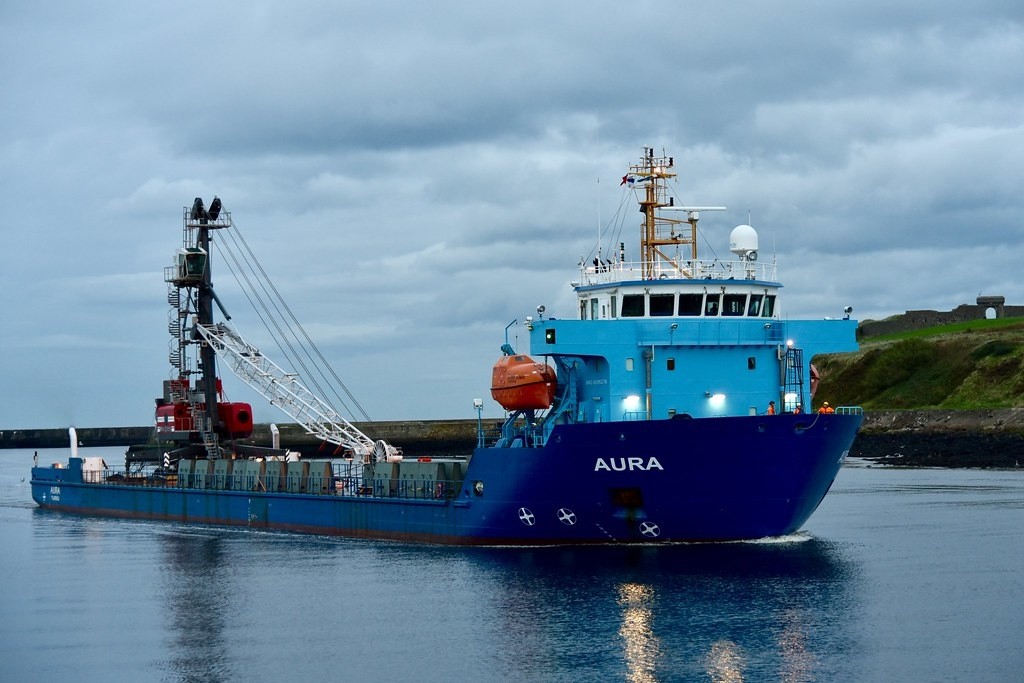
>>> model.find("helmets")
[823,402,829,405]
[796,403,801,406]
[769,400,775,405]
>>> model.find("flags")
[620,174,629,186]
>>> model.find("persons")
[817,401,834,414]
[768,401,775,415]
[793,403,801,415]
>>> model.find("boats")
[28,142,867,545]
[489,353,558,408]
